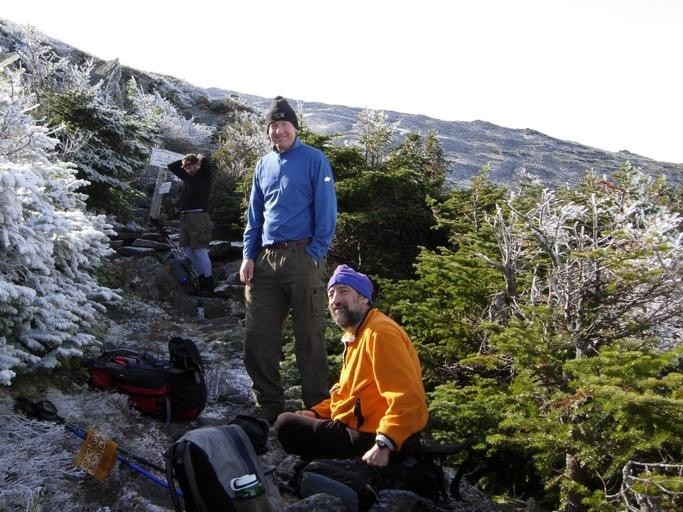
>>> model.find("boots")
[193,274,212,296]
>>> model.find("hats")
[327,264,373,304]
[266,96,297,134]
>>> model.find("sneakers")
[257,405,282,425]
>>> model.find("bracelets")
[376,439,385,449]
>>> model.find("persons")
[274,262,429,478]
[237,95,338,429]
[167,153,215,298]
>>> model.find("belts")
[266,237,312,249]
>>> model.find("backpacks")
[163,414,283,512]
[280,459,379,512]
[81,337,207,422]
